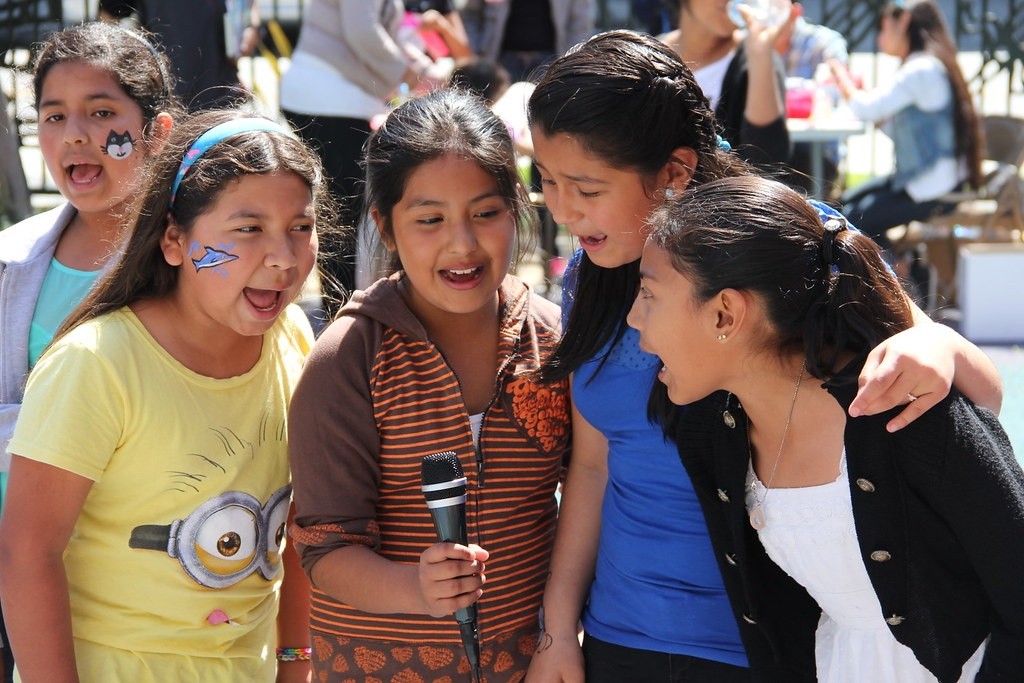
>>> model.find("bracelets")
[274,648,312,662]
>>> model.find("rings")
[908,394,917,401]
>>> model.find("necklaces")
[746,358,805,530]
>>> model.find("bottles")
[810,63,835,131]
[724,0,792,29]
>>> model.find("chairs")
[892,102,1024,322]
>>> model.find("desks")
[788,108,865,203]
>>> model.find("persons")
[0,110,326,683]
[524,30,1001,683]
[0,22,175,481]
[628,179,1024,683]
[291,91,571,683]
[98,0,979,320]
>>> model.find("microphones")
[420,450,482,667]
